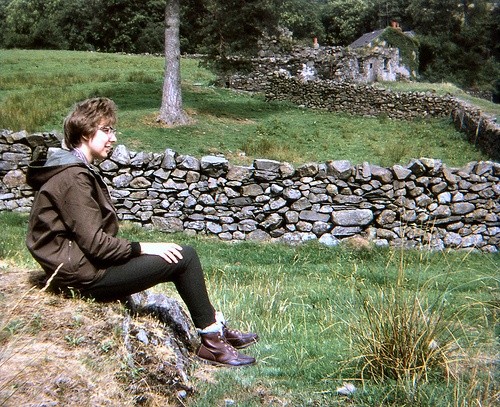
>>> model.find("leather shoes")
[221,320,260,349]
[195,326,257,367]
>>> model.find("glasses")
[93,125,117,134]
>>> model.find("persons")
[26,98,259,369]
